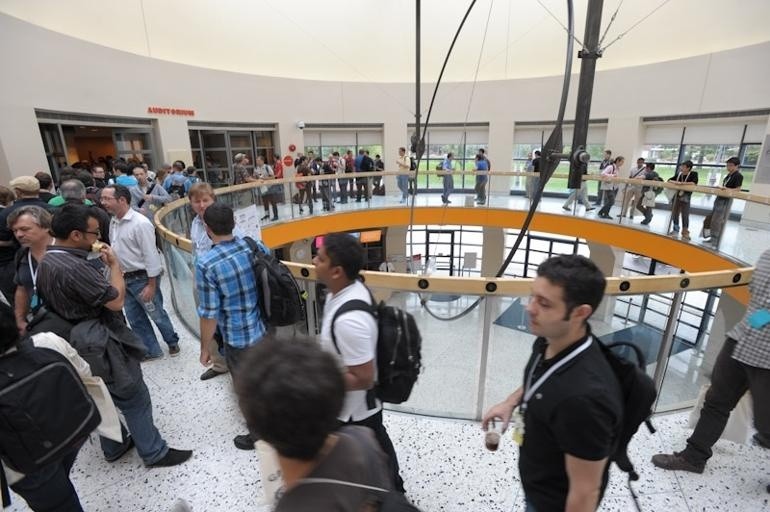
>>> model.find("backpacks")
[340,425,422,512]
[0,336,102,476]
[86,187,100,206]
[404,156,417,171]
[166,174,189,199]
[242,235,307,328]
[594,334,659,481]
[330,281,422,411]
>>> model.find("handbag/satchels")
[25,304,70,344]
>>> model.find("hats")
[234,153,246,162]
[9,174,41,193]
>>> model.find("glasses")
[81,230,101,239]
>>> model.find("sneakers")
[260,196,372,222]
[652,451,705,475]
[474,195,486,205]
[399,195,409,205]
[200,369,227,381]
[561,205,720,247]
[441,195,452,205]
[147,447,193,468]
[105,435,135,464]
[168,345,180,357]
[233,435,255,451]
[139,354,164,363]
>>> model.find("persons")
[1,301,85,511]
[651,248,770,494]
[36,202,193,468]
[396,147,411,203]
[472,154,488,205]
[441,152,455,203]
[522,150,664,225]
[379,258,395,273]
[482,254,626,512]
[667,159,698,242]
[1,147,384,380]
[312,232,407,498]
[479,149,491,171]
[409,157,417,195]
[196,201,273,451]
[234,335,421,511]
[702,156,743,251]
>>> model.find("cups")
[483,426,504,450]
[107,178,114,186]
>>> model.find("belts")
[123,270,146,280]
